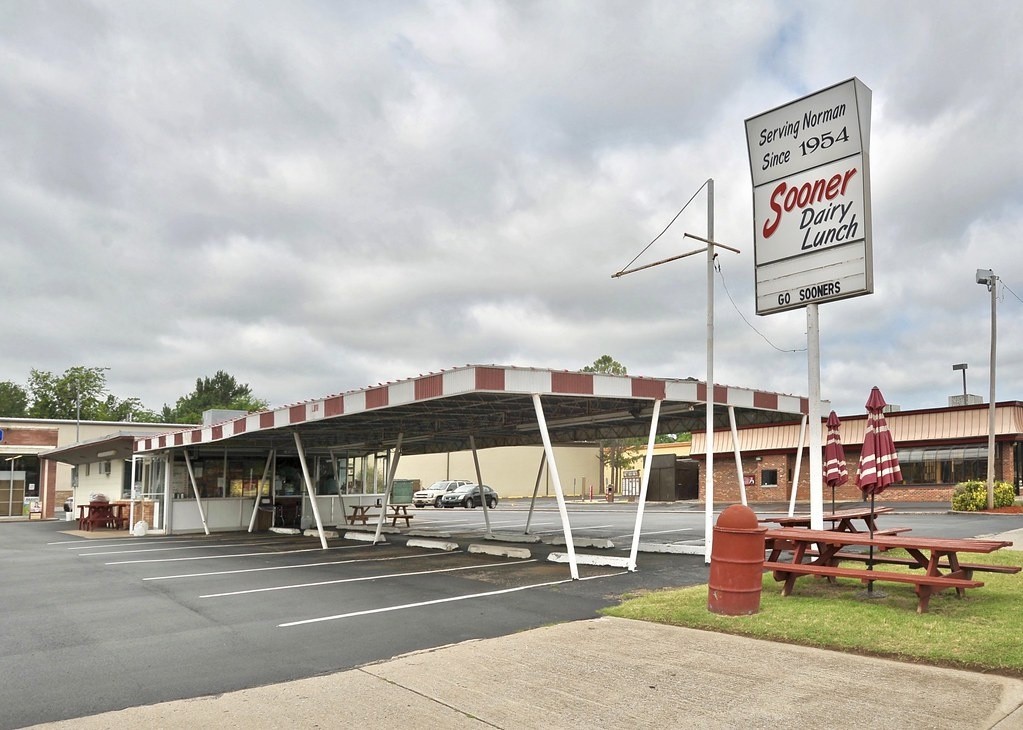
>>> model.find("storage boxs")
[30,513,42,519]
[230,480,258,497]
[30,502,42,512]
[258,479,270,496]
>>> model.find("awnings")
[894,442,1000,463]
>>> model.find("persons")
[34,502,40,508]
[215,487,223,496]
[283,479,295,494]
[194,484,208,498]
[323,475,340,494]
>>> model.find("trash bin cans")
[707,501,770,618]
[390,479,414,505]
[608,484,614,502]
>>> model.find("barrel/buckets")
[707,525,765,616]
[134,521,148,537]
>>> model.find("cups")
[377,499,382,505]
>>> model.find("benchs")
[76,518,128,532]
[874,528,913,535]
[345,514,414,520]
[789,549,1022,582]
[762,562,985,588]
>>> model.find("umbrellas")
[822,409,849,530]
[855,385,903,593]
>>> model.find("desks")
[77,504,127,532]
[758,506,1023,613]
[349,504,411,528]
